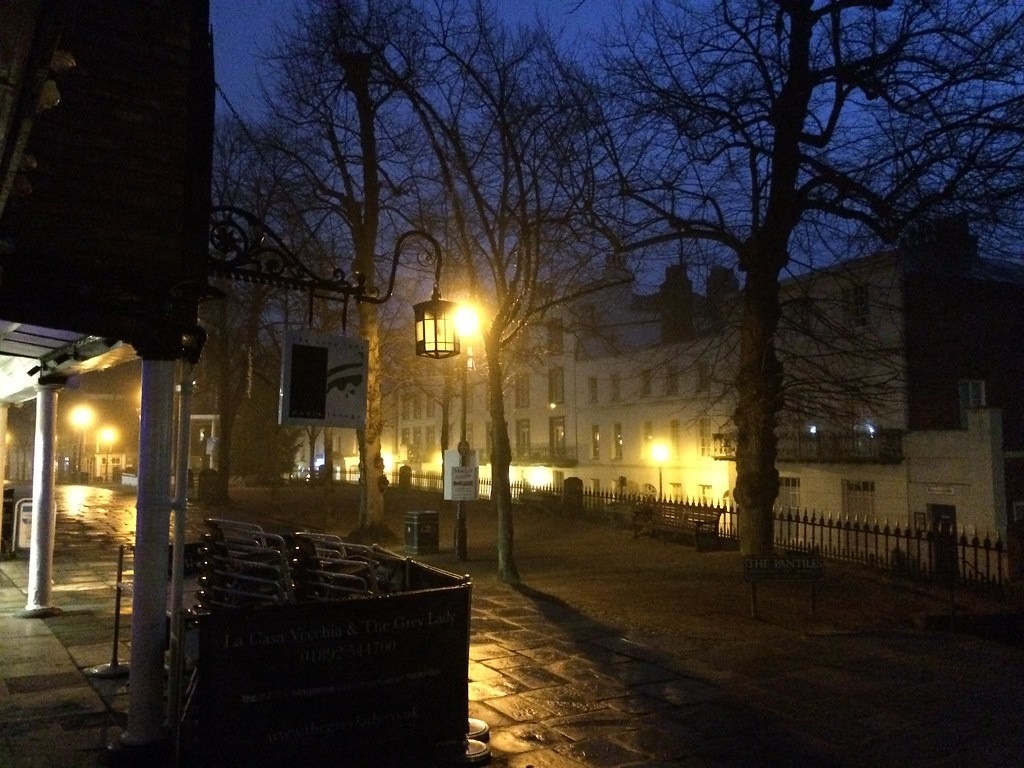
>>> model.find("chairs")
[185,516,378,623]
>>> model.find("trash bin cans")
[403,511,440,554]
[198,469,218,503]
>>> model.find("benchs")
[630,501,725,552]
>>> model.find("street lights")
[75,412,91,482]
[449,296,484,570]
[103,428,116,483]
[650,443,667,502]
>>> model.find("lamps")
[207,206,463,360]
